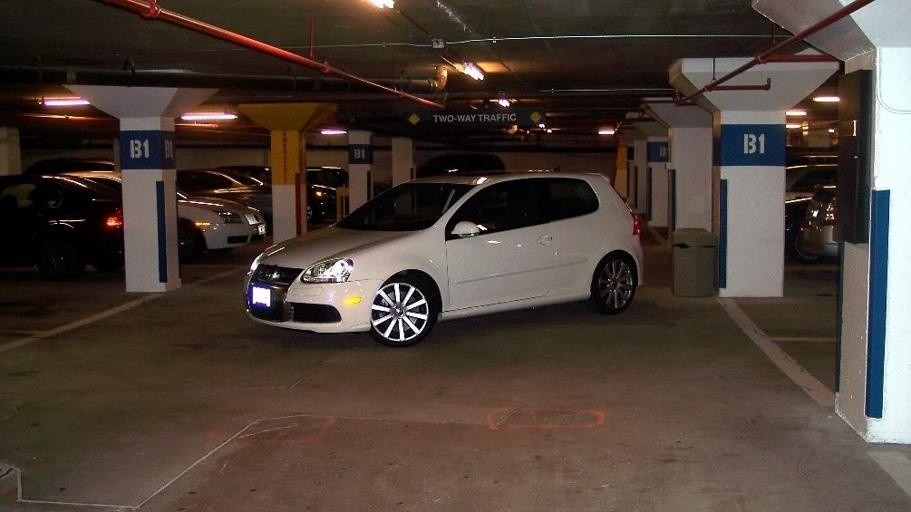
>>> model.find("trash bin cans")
[671,227,716,297]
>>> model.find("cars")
[243,171,646,347]
[177,167,315,230]
[23,157,266,265]
[784,163,835,244]
[0,171,205,283]
[798,184,840,266]
[221,164,339,227]
